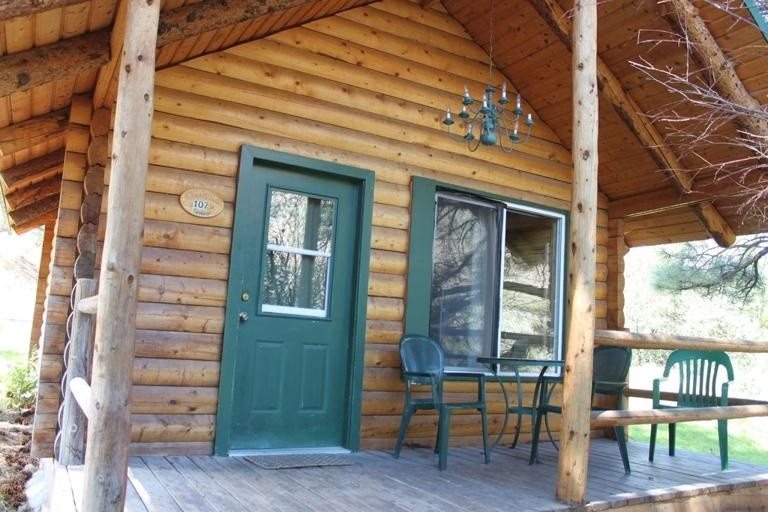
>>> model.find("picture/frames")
[649,350,734,472]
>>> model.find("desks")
[476,357,565,464]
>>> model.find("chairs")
[528,345,632,474]
[393,334,491,470]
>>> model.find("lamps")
[443,0,534,152]
